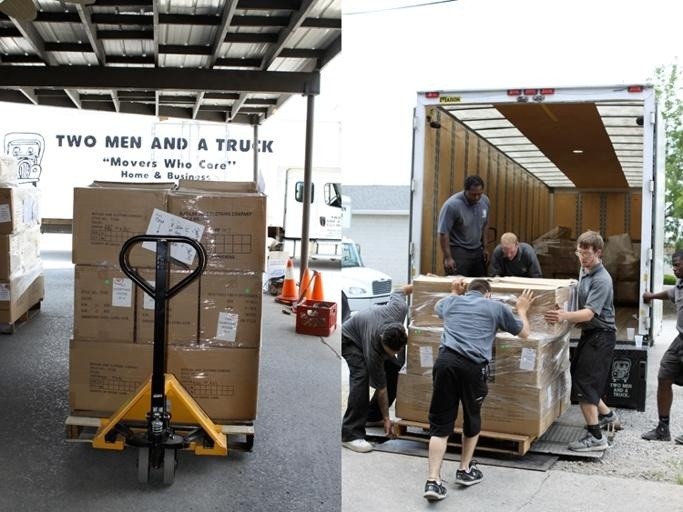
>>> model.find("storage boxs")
[395,271,580,437]
[0,188,45,324]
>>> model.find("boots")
[641,421,670,441]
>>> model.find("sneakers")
[584,409,620,429]
[341,439,373,452]
[365,417,395,427]
[568,432,609,451]
[454,460,483,486]
[423,479,448,500]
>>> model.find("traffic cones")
[311,270,324,302]
[291,268,310,313]
[275,257,297,306]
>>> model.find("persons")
[544,230,617,453]
[490,233,543,279]
[437,175,491,277]
[342,273,437,454]
[642,251,683,444]
[424,279,537,502]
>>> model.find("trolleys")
[65,232,255,488]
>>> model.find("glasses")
[575,251,593,258]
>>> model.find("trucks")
[408,78,669,411]
[1,93,351,252]
[272,236,395,323]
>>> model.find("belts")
[594,327,615,332]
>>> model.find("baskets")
[296,299,337,336]
